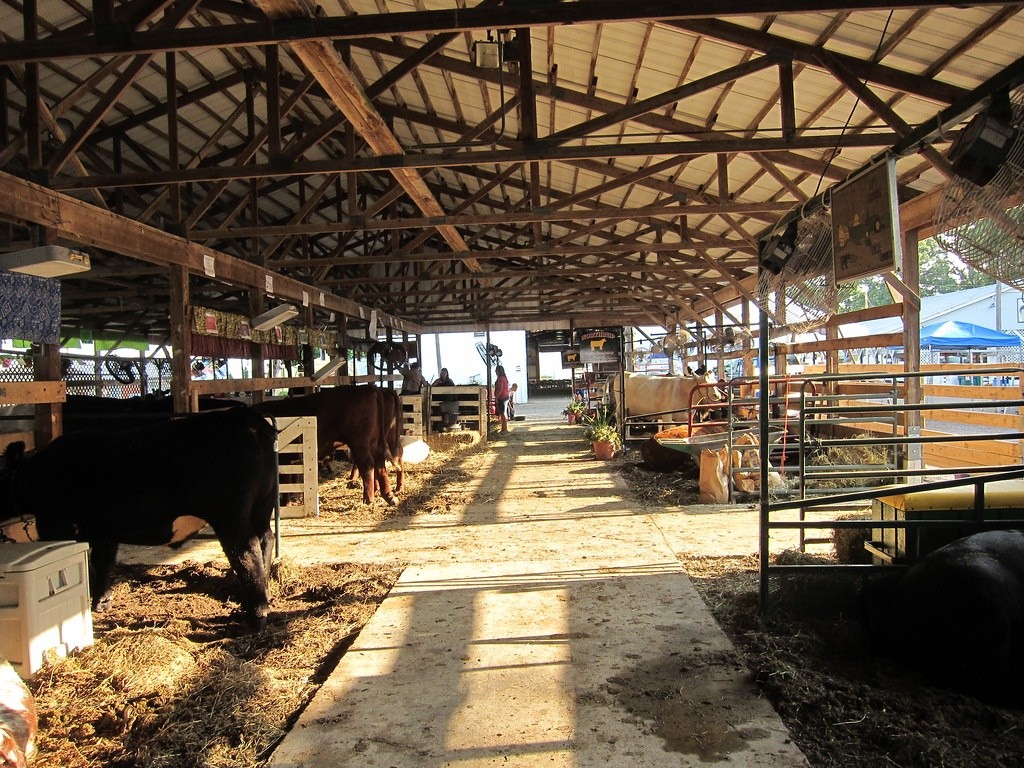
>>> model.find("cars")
[703,353,806,397]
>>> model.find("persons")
[432,368,455,386]
[395,361,430,396]
[0,356,11,370]
[507,382,518,421]
[191,362,211,381]
[495,365,510,435]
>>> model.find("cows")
[0,406,279,635]
[848,527,1024,709]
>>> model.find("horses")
[250,384,399,505]
[346,387,404,492]
[613,372,722,439]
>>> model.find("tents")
[885,321,1023,413]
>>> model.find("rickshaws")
[655,425,810,469]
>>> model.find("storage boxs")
[0,541,94,678]
